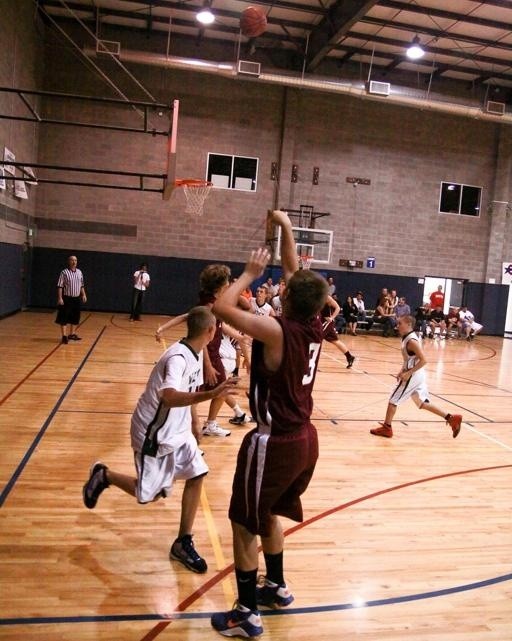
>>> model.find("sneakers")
[202,423,231,437]
[171,533,207,573]
[370,421,392,437]
[211,602,263,637]
[253,579,294,606]
[346,357,355,368]
[82,462,108,509]
[229,413,251,424]
[447,415,462,437]
[68,334,81,340]
[62,336,68,344]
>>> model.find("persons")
[155,265,251,437]
[83,305,237,575]
[129,265,151,323]
[57,256,87,343]
[230,277,342,318]
[374,285,485,341]
[342,290,366,336]
[318,295,355,368]
[370,314,462,437]
[211,210,330,640]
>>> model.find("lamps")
[405,35,425,60]
[196,0,215,25]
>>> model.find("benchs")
[338,307,458,336]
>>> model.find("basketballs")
[240,6,267,37]
